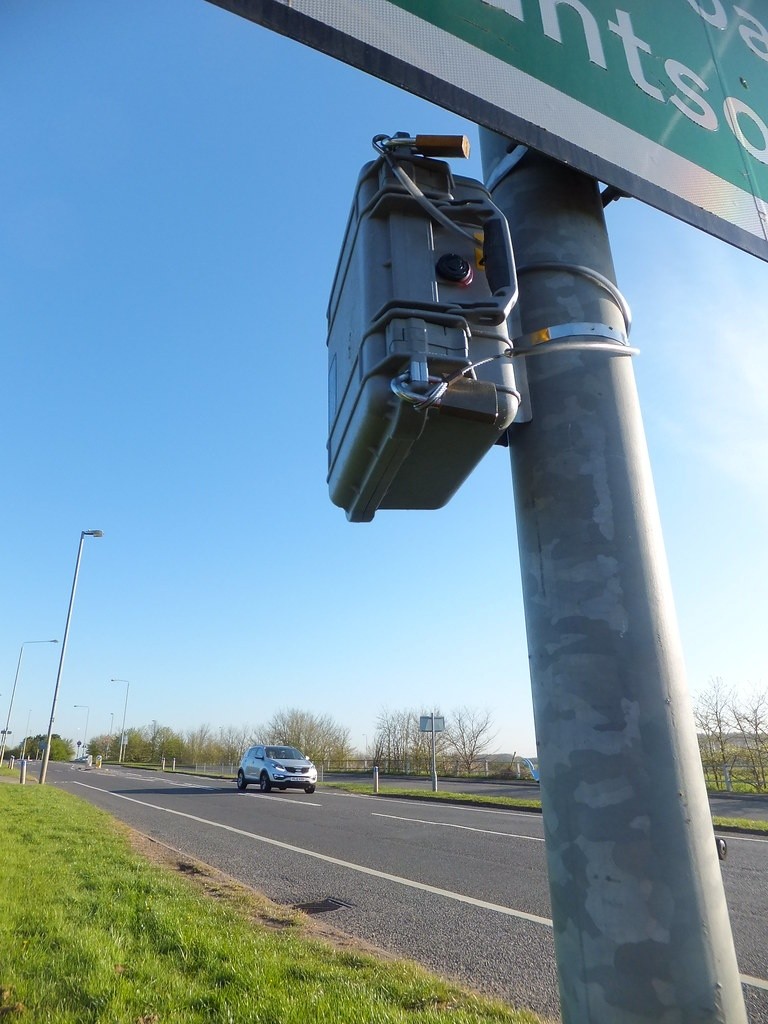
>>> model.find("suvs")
[238,744,317,794]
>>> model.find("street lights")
[153,719,156,735]
[39,529,104,784]
[110,713,114,737]
[22,709,32,761]
[0,639,58,767]
[74,705,89,758]
[111,679,129,762]
